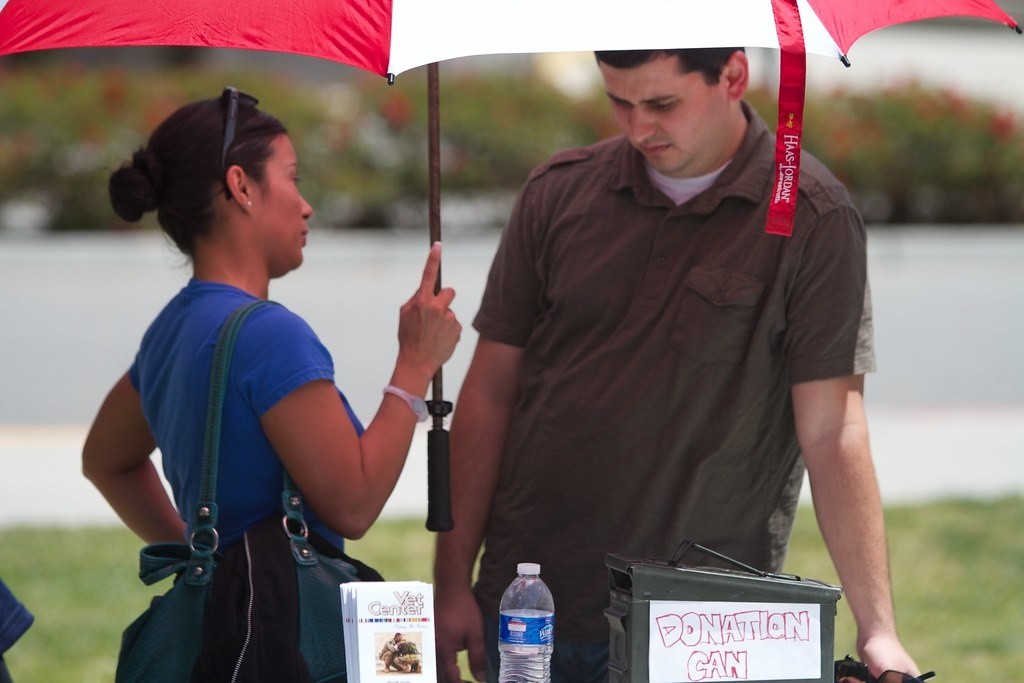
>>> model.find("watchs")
[380,382,430,425]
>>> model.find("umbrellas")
[0,0,1021,530]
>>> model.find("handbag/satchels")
[113,301,383,683]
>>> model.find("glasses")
[834,656,935,683]
[220,87,259,201]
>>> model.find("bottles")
[498,563,555,683]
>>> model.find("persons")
[81,86,461,579]
[430,48,926,683]
[378,633,421,673]
